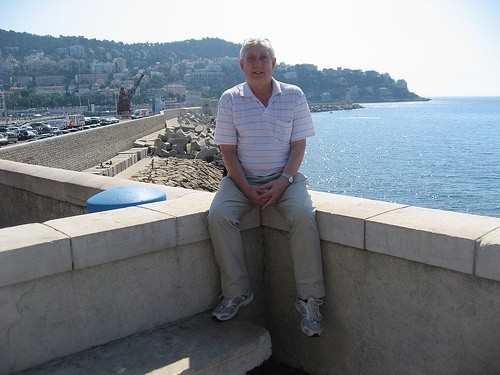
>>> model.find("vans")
[131,109,149,119]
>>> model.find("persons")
[207,37,326,337]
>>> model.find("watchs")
[281,172,293,184]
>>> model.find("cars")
[0,114,120,146]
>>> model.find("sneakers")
[211,290,254,321]
[295,297,323,336]
